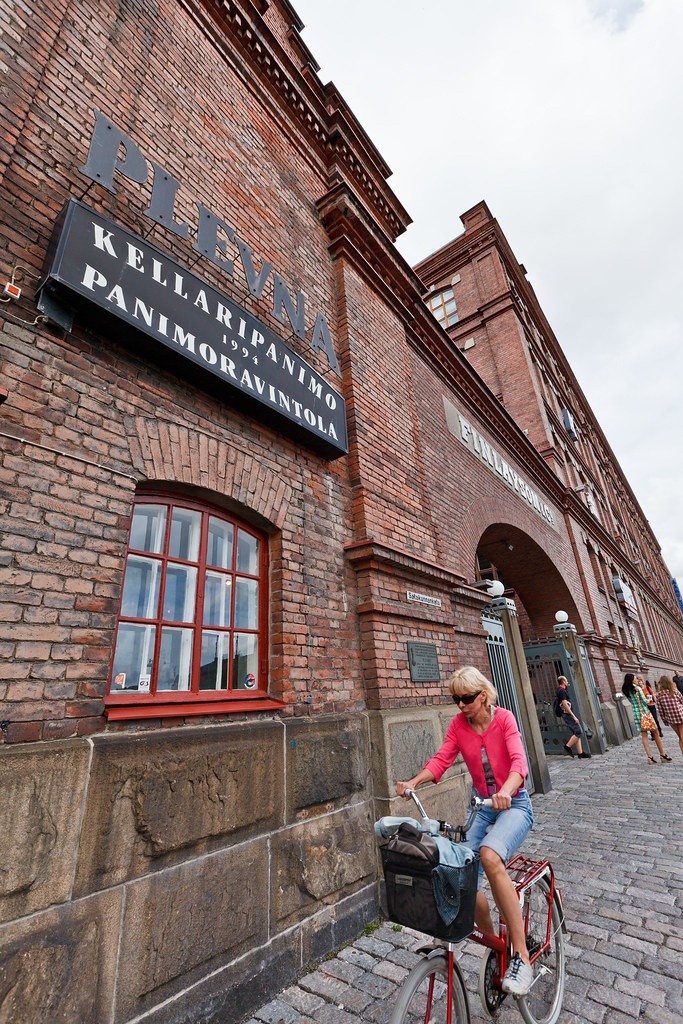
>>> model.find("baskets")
[379,833,481,944]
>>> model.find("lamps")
[555,608,569,622]
[488,579,505,598]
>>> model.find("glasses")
[452,691,481,704]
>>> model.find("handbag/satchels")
[386,823,442,932]
[640,713,658,732]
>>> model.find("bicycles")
[371,788,567,1024]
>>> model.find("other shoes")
[578,751,591,758]
[563,744,574,758]
[651,737,654,741]
[660,732,663,738]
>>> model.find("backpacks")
[552,688,563,717]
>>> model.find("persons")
[395,666,534,997]
[622,670,683,765]
[556,676,591,759]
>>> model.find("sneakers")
[501,952,534,996]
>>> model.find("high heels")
[648,757,657,765]
[660,754,672,763]
